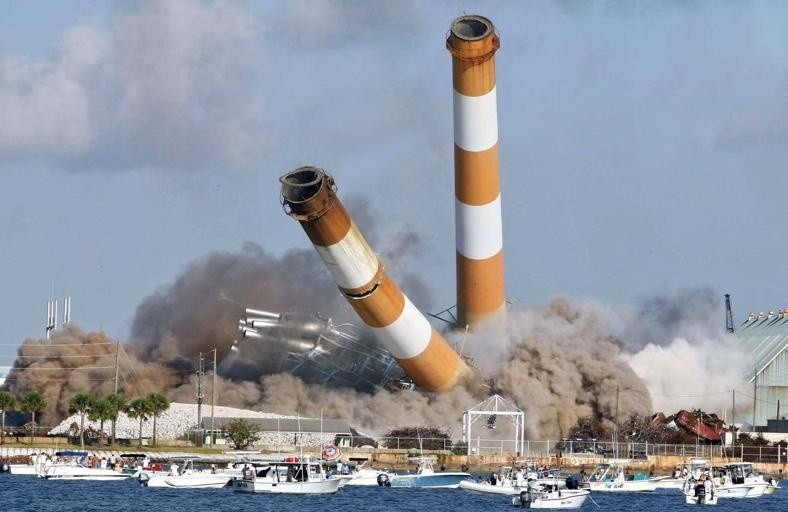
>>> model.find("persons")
[226,461,234,469]
[241,464,249,477]
[469,463,548,486]
[779,469,784,480]
[341,462,349,473]
[771,477,776,485]
[440,464,446,472]
[416,467,418,473]
[336,460,343,472]
[28,451,156,474]
[595,472,600,481]
[583,472,588,482]
[212,464,216,474]
[272,471,278,484]
[170,462,179,476]
[579,471,583,477]
[695,480,705,505]
[671,466,737,484]
[558,491,562,498]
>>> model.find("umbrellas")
[322,445,341,462]
